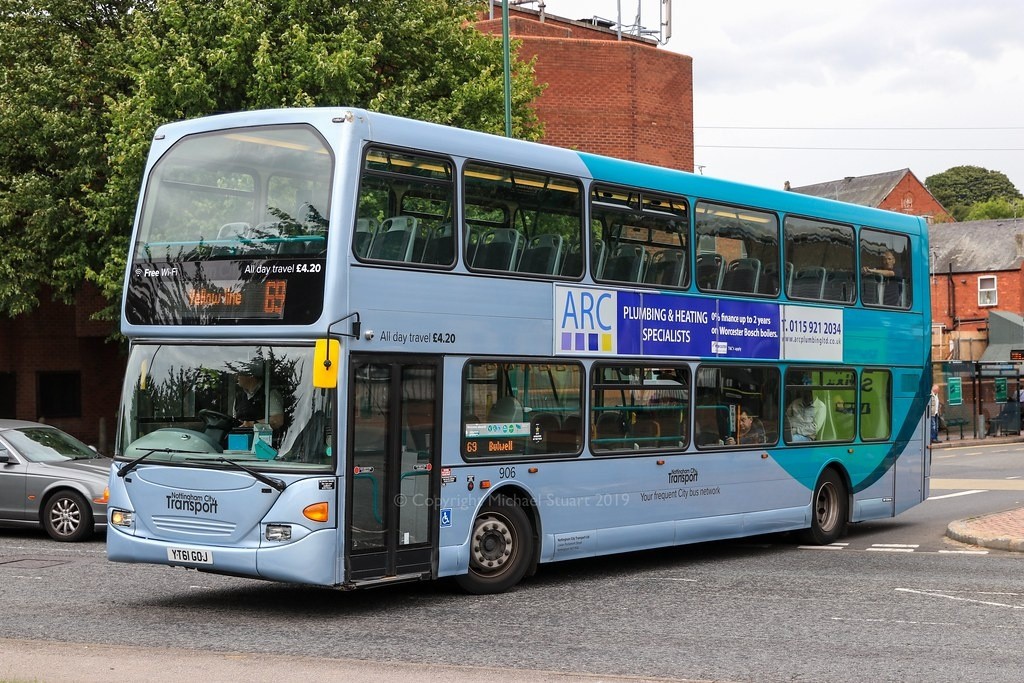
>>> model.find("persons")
[930,384,942,443]
[725,404,764,445]
[229,357,284,432]
[463,415,481,443]
[1012,383,1024,431]
[787,380,827,442]
[861,249,903,305]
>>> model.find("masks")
[1006,399,1011,402]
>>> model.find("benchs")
[944,418,969,441]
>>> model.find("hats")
[233,363,264,376]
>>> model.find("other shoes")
[932,439,942,443]
[985,432,996,437]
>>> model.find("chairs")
[567,238,606,279]
[575,245,609,280]
[538,240,569,275]
[643,249,686,286]
[695,253,724,290]
[487,388,838,452]
[355,217,378,256]
[657,255,689,286]
[862,272,885,305]
[698,258,728,289]
[601,243,646,283]
[518,233,564,276]
[468,229,481,268]
[636,250,651,284]
[422,222,470,265]
[825,270,855,301]
[208,220,327,260]
[474,228,520,272]
[760,262,794,296]
[510,233,527,272]
[409,224,430,263]
[721,257,762,293]
[794,265,826,299]
[897,278,909,308]
[368,215,418,262]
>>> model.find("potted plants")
[986,291,991,304]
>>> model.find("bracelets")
[253,420,259,425]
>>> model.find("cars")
[0,418,114,542]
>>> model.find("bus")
[103,104,934,597]
